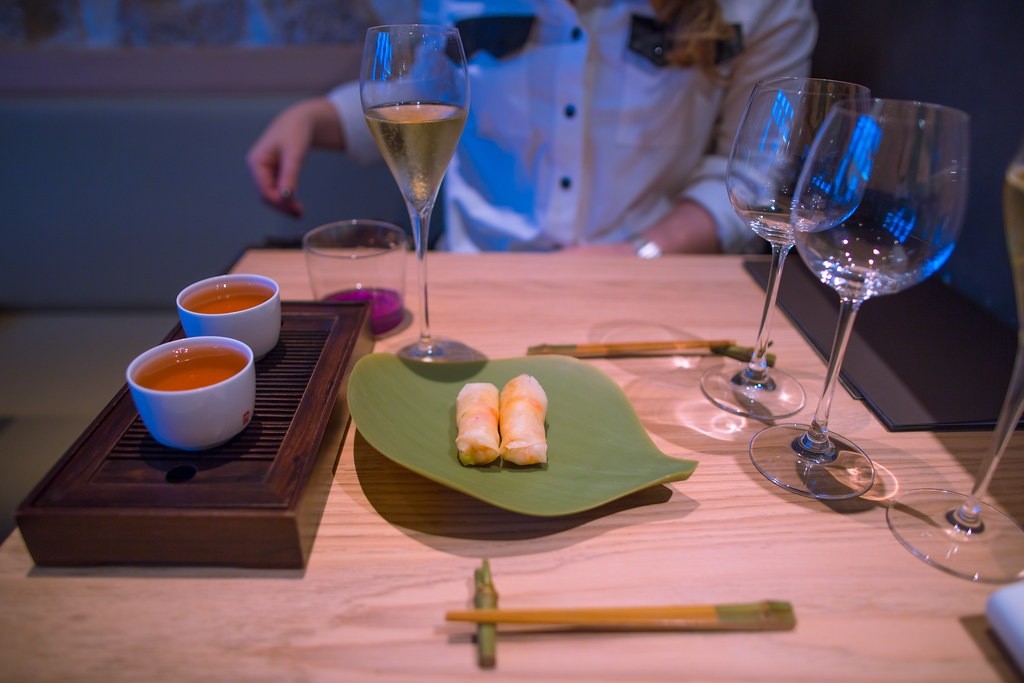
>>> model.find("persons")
[249,0,821,257]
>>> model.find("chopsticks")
[528,338,737,357]
[445,600,796,629]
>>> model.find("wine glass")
[701,74,870,421]
[750,99,968,498]
[887,155,1024,583]
[360,25,476,364]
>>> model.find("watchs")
[630,236,660,259]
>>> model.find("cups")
[177,274,282,363]
[304,221,410,335]
[127,336,255,451]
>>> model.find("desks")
[0,239,1024,683]
